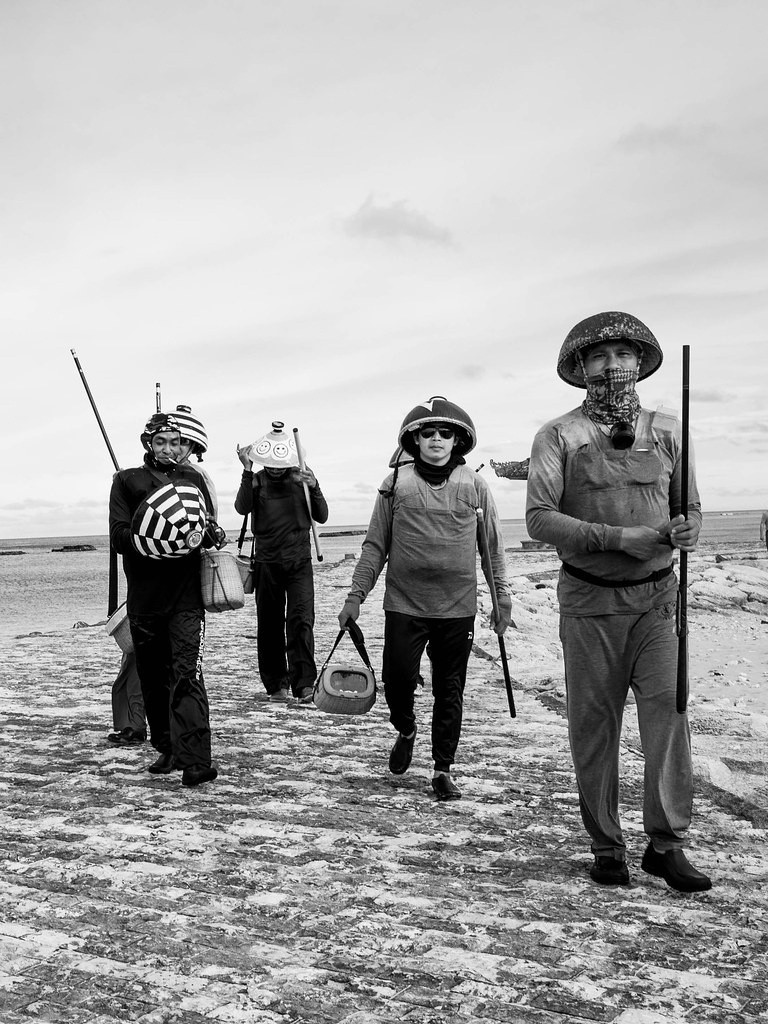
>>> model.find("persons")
[527,311,712,892]
[760,510,768,549]
[337,399,512,800]
[109,413,226,784]
[235,420,328,701]
[107,405,218,744]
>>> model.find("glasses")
[420,425,457,440]
[180,438,191,445]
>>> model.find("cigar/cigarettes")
[169,458,177,465]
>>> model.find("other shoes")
[270,687,288,701]
[298,687,314,704]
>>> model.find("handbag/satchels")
[201,550,244,612]
[236,555,253,594]
[106,602,134,653]
[313,618,377,714]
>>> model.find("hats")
[165,405,208,453]
[556,311,661,389]
[245,421,306,468]
[142,412,181,438]
[398,400,476,458]
[130,481,207,560]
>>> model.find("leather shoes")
[108,727,144,744]
[642,841,712,891]
[432,774,461,799]
[389,725,417,774]
[589,855,631,885]
[148,754,175,773]
[182,767,218,786]
